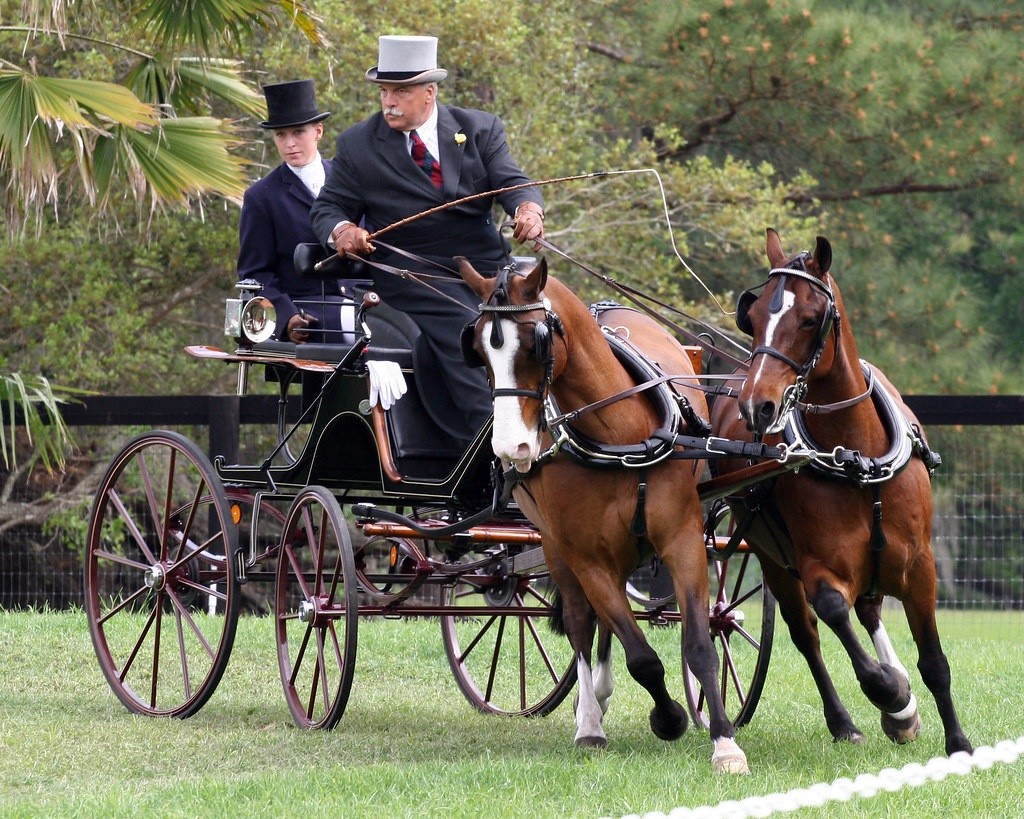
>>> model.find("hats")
[257,79,331,130]
[365,35,448,85]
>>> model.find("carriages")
[82,226,990,784]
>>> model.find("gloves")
[510,202,544,254]
[365,360,407,411]
[332,223,376,260]
[286,314,319,344]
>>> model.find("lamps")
[225,279,276,397]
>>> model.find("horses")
[450,226,979,778]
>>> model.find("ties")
[409,129,442,192]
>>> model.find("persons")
[237,79,374,344]
[309,35,545,499]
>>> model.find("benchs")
[263,275,463,483]
